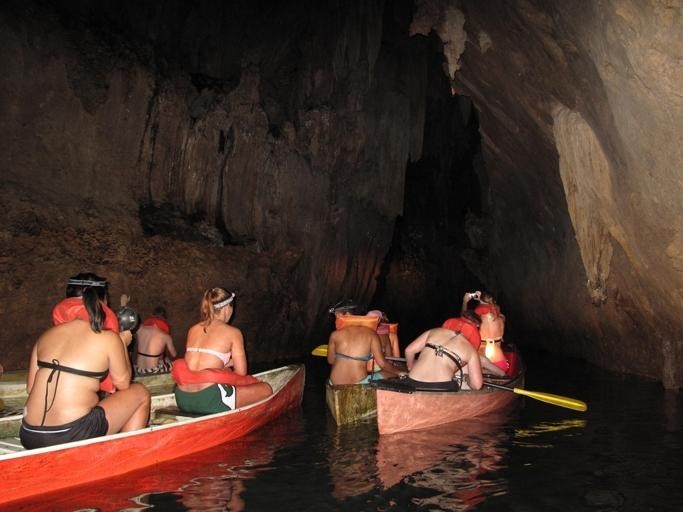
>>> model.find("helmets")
[115,307,137,331]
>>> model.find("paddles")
[312,345,407,363]
[454,375,589,412]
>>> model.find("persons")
[16,271,151,450]
[400,310,486,390]
[119,291,177,377]
[364,307,407,374]
[459,289,510,384]
[325,297,408,387]
[171,286,273,414]
